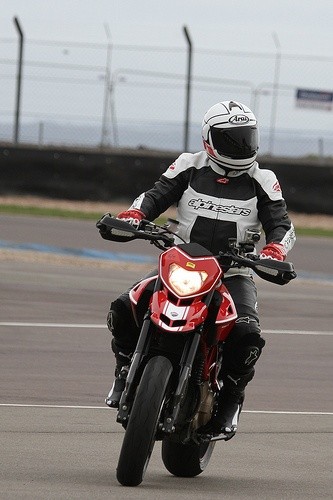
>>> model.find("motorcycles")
[95,212,297,488]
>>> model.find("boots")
[207,367,255,432]
[105,337,136,408]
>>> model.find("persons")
[105,101,296,441]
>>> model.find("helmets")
[201,100,259,170]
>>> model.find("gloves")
[117,209,147,230]
[258,241,286,262]
